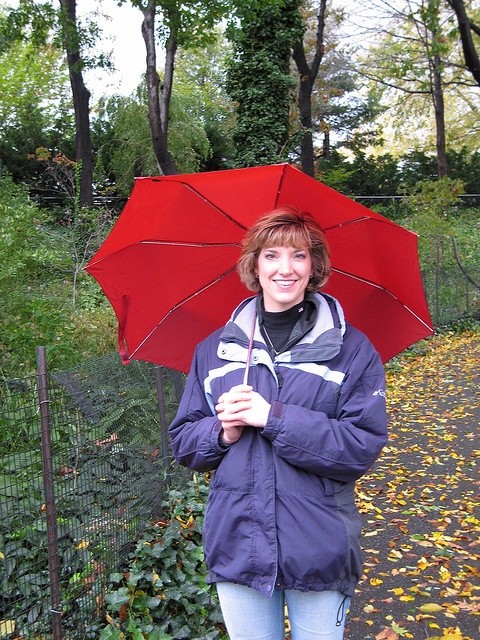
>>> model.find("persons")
[168,208,390,640]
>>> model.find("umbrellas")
[80,159,437,427]
